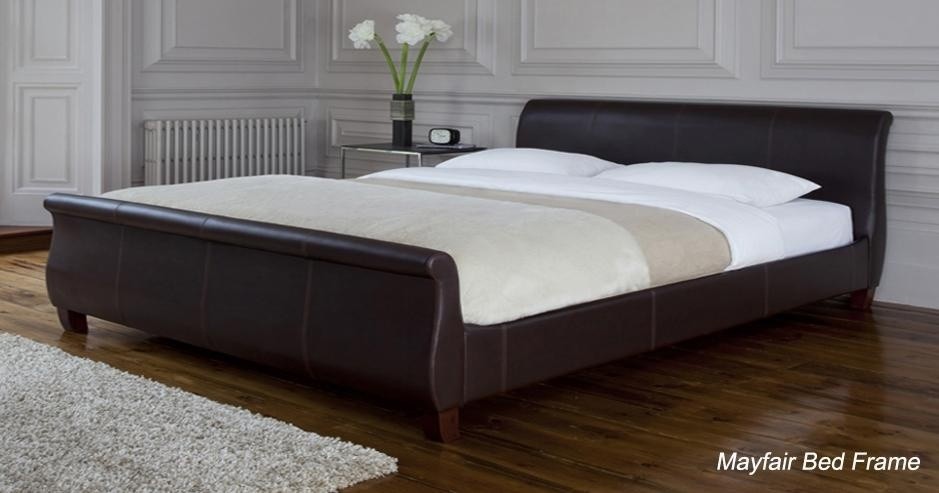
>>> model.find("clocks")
[429,128,460,146]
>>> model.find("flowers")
[348,13,454,94]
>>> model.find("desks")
[341,142,486,178]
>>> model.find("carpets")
[0,330,399,493]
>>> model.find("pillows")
[435,147,621,179]
[597,162,821,208]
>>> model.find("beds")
[44,98,893,444]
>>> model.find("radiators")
[144,117,306,184]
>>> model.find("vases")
[390,94,415,147]
